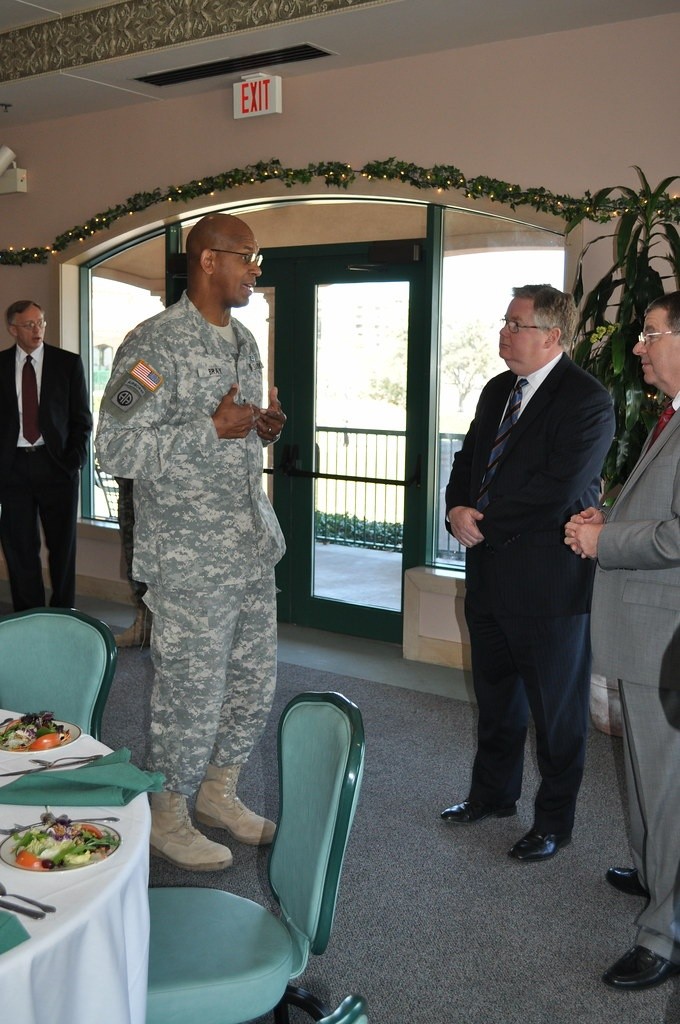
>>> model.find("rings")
[268,428,272,432]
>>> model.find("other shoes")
[114,610,150,648]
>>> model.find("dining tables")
[0,708,168,1024]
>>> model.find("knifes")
[0,756,102,777]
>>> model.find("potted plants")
[568,164,680,739]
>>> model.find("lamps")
[0,145,29,193]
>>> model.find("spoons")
[0,883,56,912]
[29,755,103,766]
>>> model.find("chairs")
[0,606,120,743]
[145,689,368,1024]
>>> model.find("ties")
[476,378,529,512]
[645,401,676,454]
[22,355,41,444]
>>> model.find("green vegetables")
[13,817,119,866]
[0,711,69,746]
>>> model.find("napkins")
[0,746,168,807]
[0,909,31,955]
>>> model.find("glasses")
[638,331,671,346]
[500,319,539,333]
[211,249,264,266]
[15,321,47,329]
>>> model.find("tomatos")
[29,733,61,750]
[81,824,101,837]
[17,850,45,869]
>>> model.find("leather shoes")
[606,867,648,897]
[440,798,516,824]
[509,827,572,861]
[602,945,677,991]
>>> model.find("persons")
[439,284,616,863]
[0,300,94,612]
[563,287,680,991]
[96,212,291,873]
[113,476,152,647]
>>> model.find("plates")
[0,719,83,753]
[0,823,121,872]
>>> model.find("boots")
[149,790,233,872]
[195,763,277,846]
